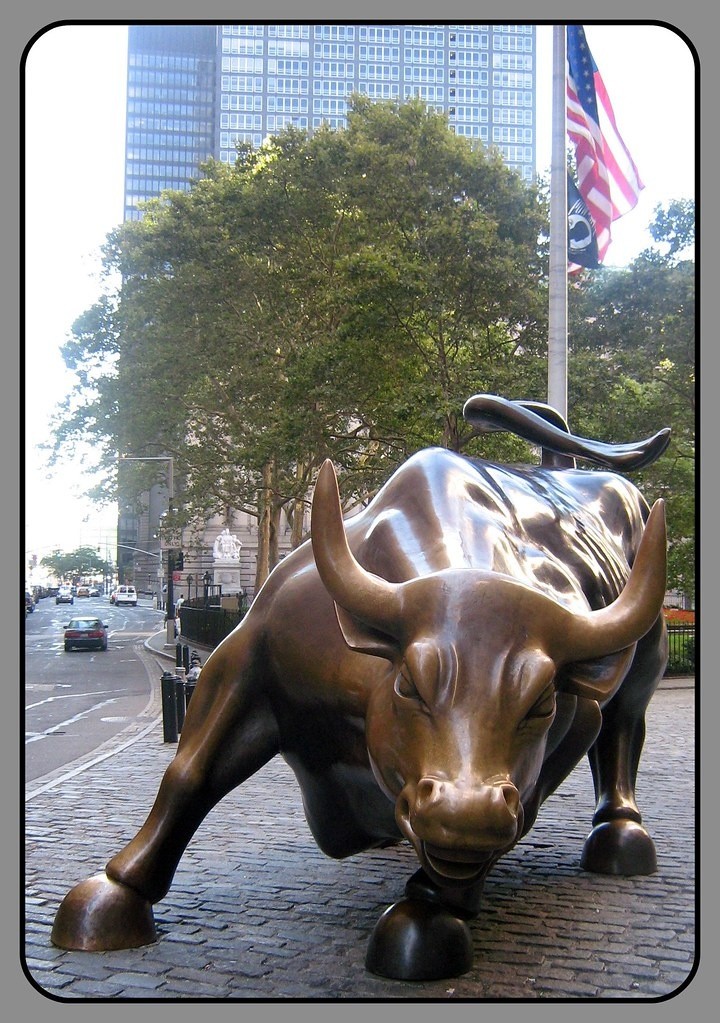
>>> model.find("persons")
[176,594,186,613]
[213,527,243,562]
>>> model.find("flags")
[562,20,644,282]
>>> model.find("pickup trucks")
[113,584,138,607]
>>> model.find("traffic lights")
[98,548,100,552]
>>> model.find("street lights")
[107,457,177,651]
[153,532,164,592]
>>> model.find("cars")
[25,584,76,610]
[90,589,100,596]
[63,616,110,650]
[110,590,116,604]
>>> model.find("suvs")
[77,587,89,597]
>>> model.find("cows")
[49,393,673,982]
